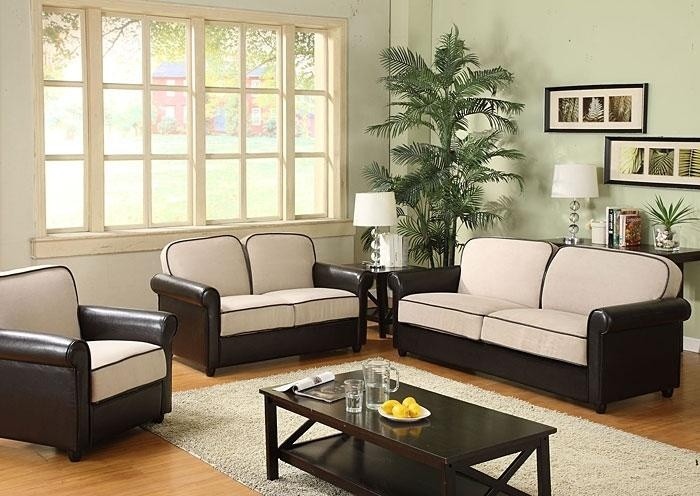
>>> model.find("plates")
[378,407,432,423]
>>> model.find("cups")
[344,379,362,413]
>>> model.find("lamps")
[353,191,397,268]
[551,164,599,243]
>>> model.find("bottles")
[361,358,400,410]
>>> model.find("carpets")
[139,355,700,496]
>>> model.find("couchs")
[388,236,691,414]
[150,232,373,377]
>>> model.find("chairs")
[0,264,179,462]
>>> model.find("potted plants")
[638,193,700,251]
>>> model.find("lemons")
[381,396,422,418]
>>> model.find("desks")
[342,263,427,339]
[539,238,700,352]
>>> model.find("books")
[272,371,367,404]
[605,206,641,248]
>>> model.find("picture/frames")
[544,83,648,133]
[604,136,700,190]
